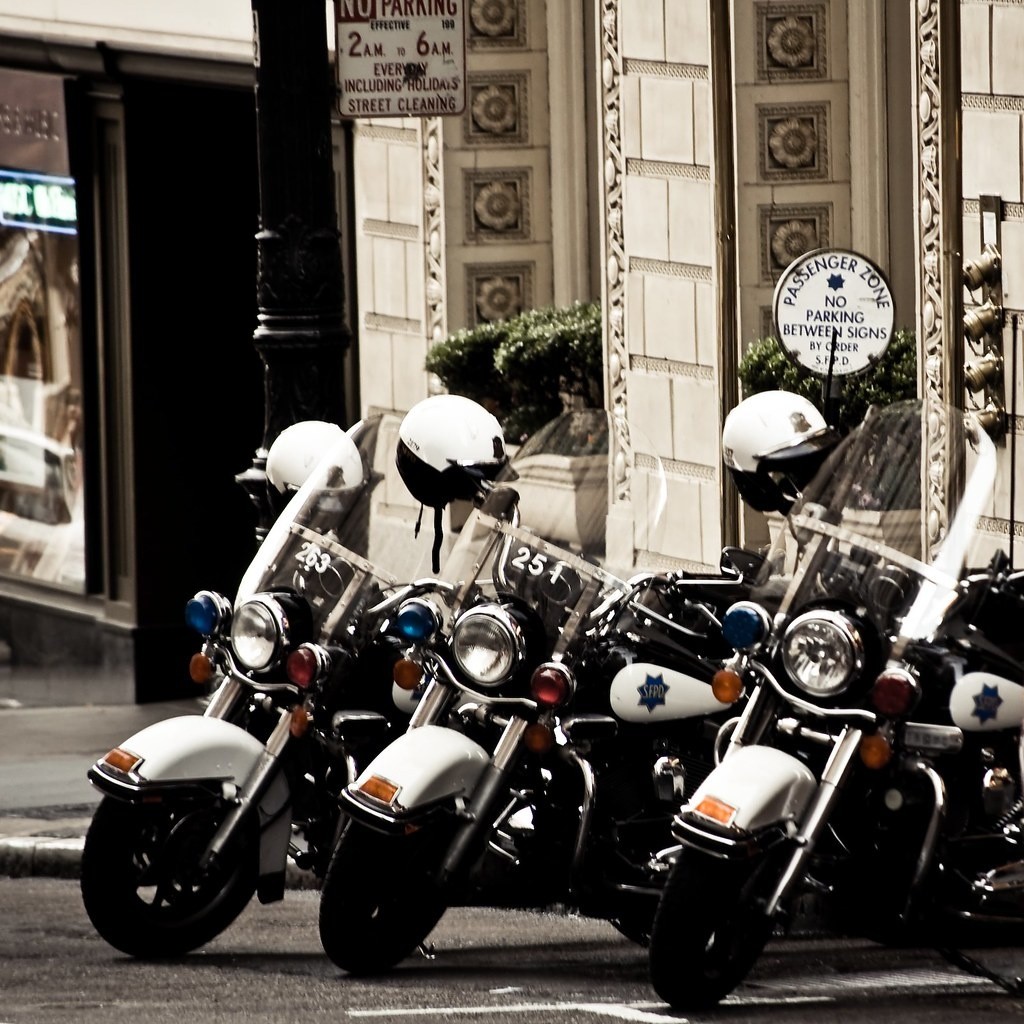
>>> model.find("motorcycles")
[73,403,481,963]
[644,396,1023,1017]
[310,389,890,977]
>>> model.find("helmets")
[723,391,841,512]
[266,419,367,495]
[396,393,520,509]
